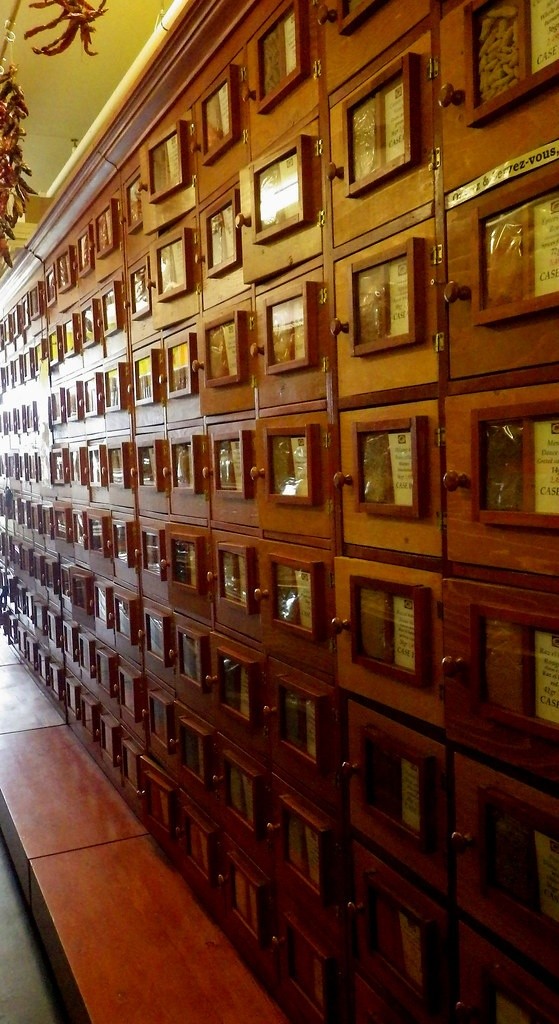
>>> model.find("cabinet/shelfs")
[0,0,559,1024]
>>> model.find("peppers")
[0,0,109,268]
